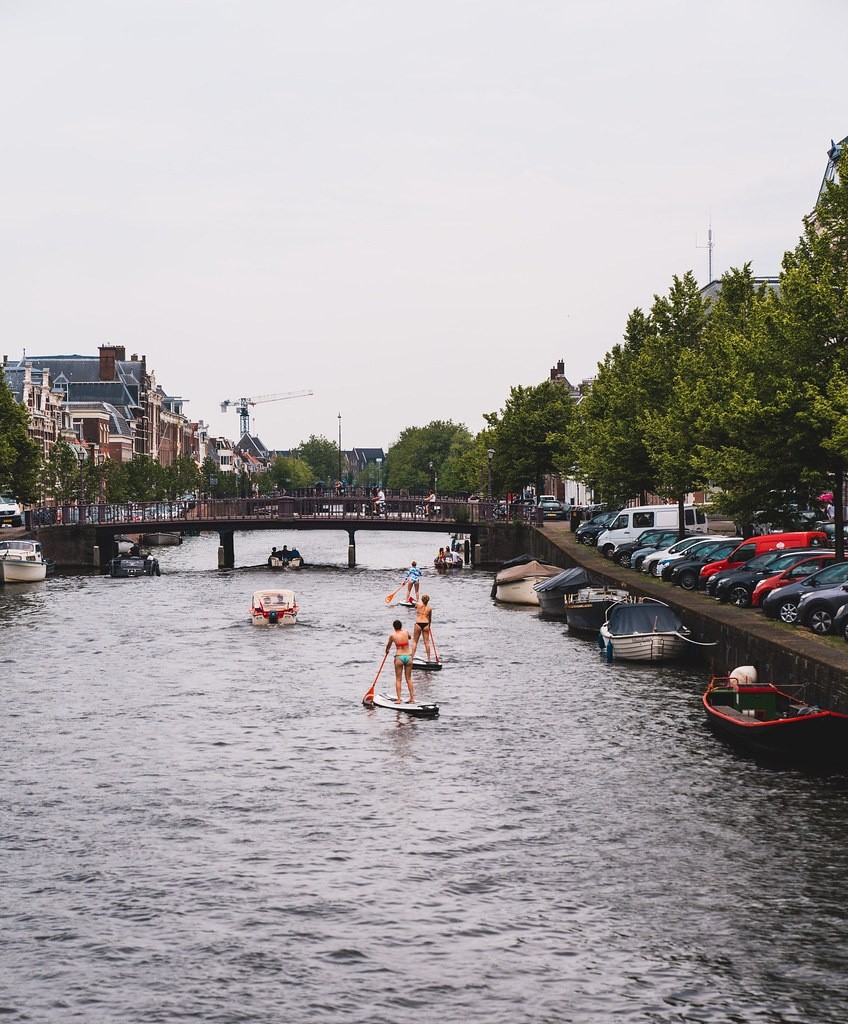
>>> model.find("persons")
[411,595,432,662]
[827,501,834,521]
[385,620,415,704]
[451,539,460,552]
[373,488,386,513]
[425,490,436,514]
[404,562,422,603]
[439,547,461,563]
[336,481,342,496]
[128,543,140,557]
[272,545,299,560]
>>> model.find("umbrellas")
[818,493,833,502]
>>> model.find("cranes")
[219,388,314,439]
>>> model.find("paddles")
[362,652,390,703]
[429,627,439,662]
[384,579,409,603]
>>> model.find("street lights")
[487,448,496,502]
[428,460,435,494]
[239,461,245,499]
[77,446,87,524]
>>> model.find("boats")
[702,669,831,753]
[490,552,566,606]
[115,534,139,553]
[142,531,182,547]
[249,588,298,627]
[563,584,644,634]
[532,565,592,618]
[600,592,692,663]
[109,552,159,579]
[0,540,56,583]
[269,551,304,572]
[434,551,463,569]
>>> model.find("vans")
[597,503,708,561]
[0,491,23,528]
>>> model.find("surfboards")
[399,599,416,607]
[373,692,439,714]
[413,656,442,669]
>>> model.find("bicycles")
[358,500,390,519]
[411,499,441,522]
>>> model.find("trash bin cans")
[591,510,603,518]
[569,510,582,532]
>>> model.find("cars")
[441,491,609,520]
[612,528,745,591]
[752,553,848,610]
[796,581,848,636]
[575,512,619,546]
[834,603,848,642]
[748,488,848,551]
[32,487,204,524]
[717,551,848,608]
[705,546,848,602]
[762,561,848,625]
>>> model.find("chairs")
[639,517,650,525]
